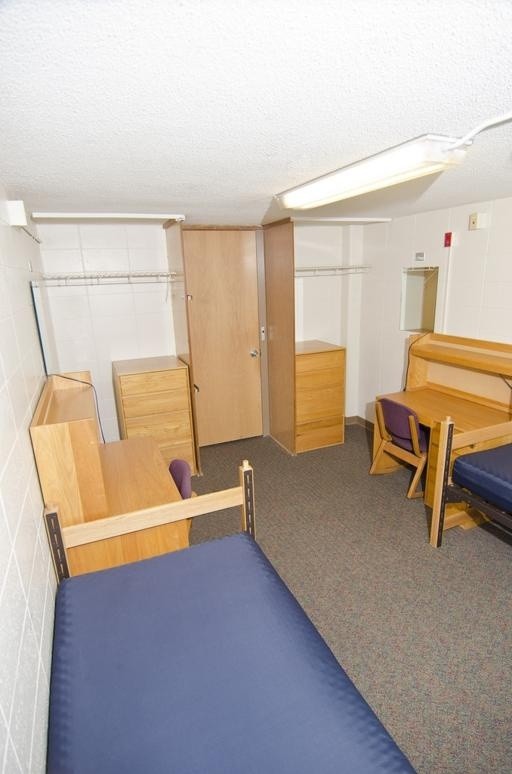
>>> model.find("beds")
[43,459,417,774]
[429,416,512,548]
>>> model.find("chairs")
[369,397,428,499]
[169,459,198,498]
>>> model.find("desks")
[28,370,188,579]
[374,332,512,531]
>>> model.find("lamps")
[272,133,461,212]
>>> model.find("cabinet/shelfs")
[112,355,198,478]
[293,339,347,455]
